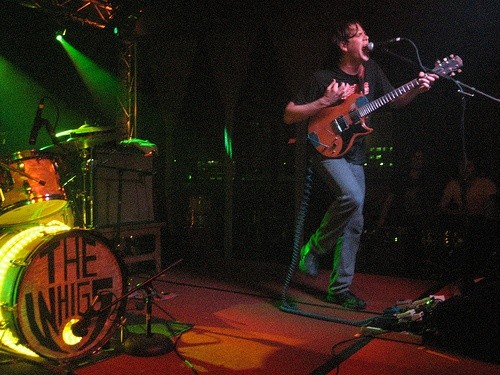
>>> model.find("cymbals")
[38,124,125,154]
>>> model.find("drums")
[0,149,69,230]
[37,201,77,227]
[0,226,127,364]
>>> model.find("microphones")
[28,97,44,144]
[368,37,401,53]
[72,290,102,335]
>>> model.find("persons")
[377,149,430,226]
[440,158,497,216]
[283,19,440,309]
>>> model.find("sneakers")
[327,289,367,309]
[299,244,319,277]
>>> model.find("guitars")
[307,52,465,158]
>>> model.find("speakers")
[424,276,500,366]
[66,148,155,226]
